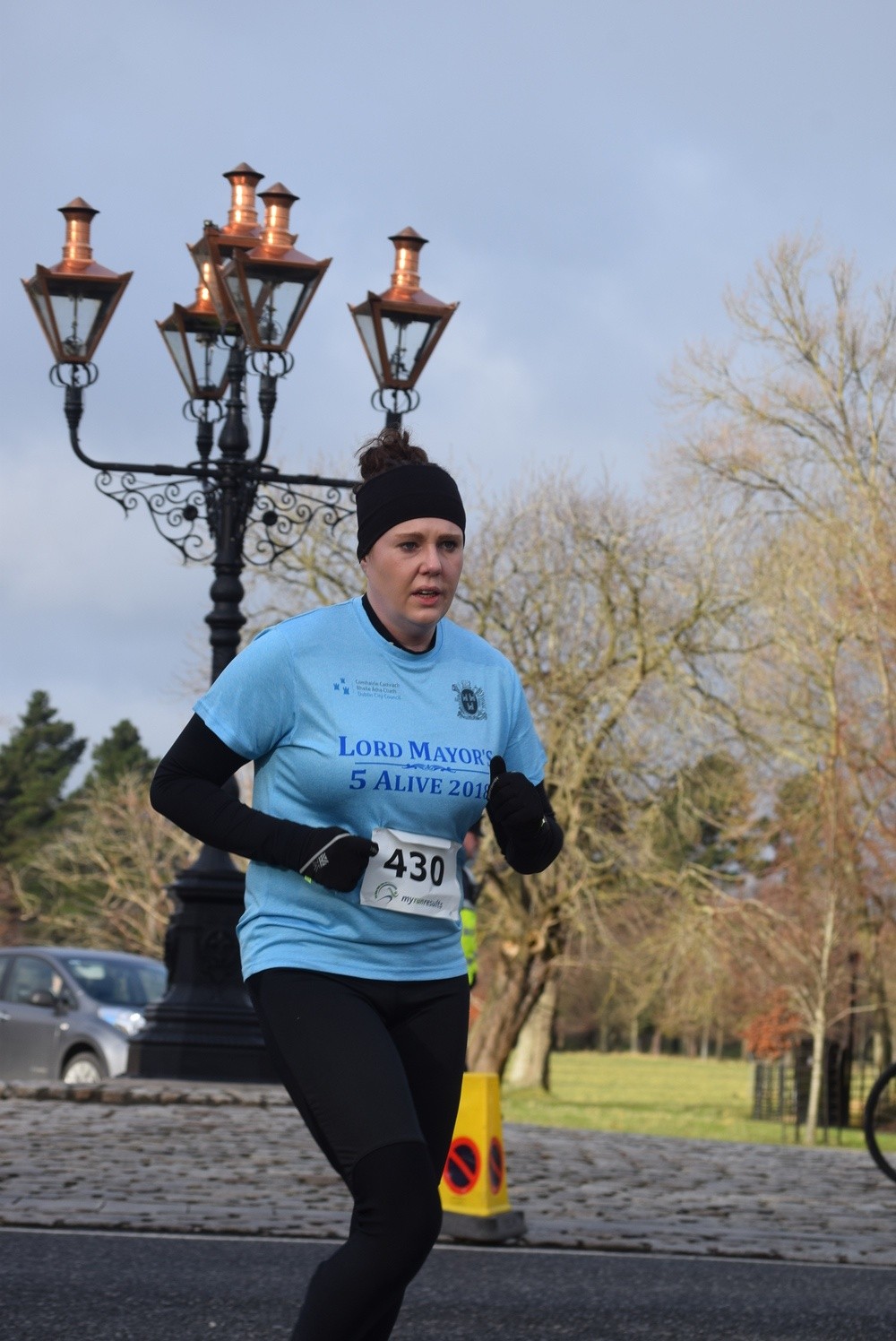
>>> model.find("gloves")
[289,824,379,892]
[486,755,543,855]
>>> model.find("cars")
[1,944,172,1092]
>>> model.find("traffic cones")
[436,1068,532,1244]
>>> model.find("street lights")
[19,158,463,1082]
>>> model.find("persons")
[147,437,565,1341]
[453,815,487,991]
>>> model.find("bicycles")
[863,1061,896,1182]
